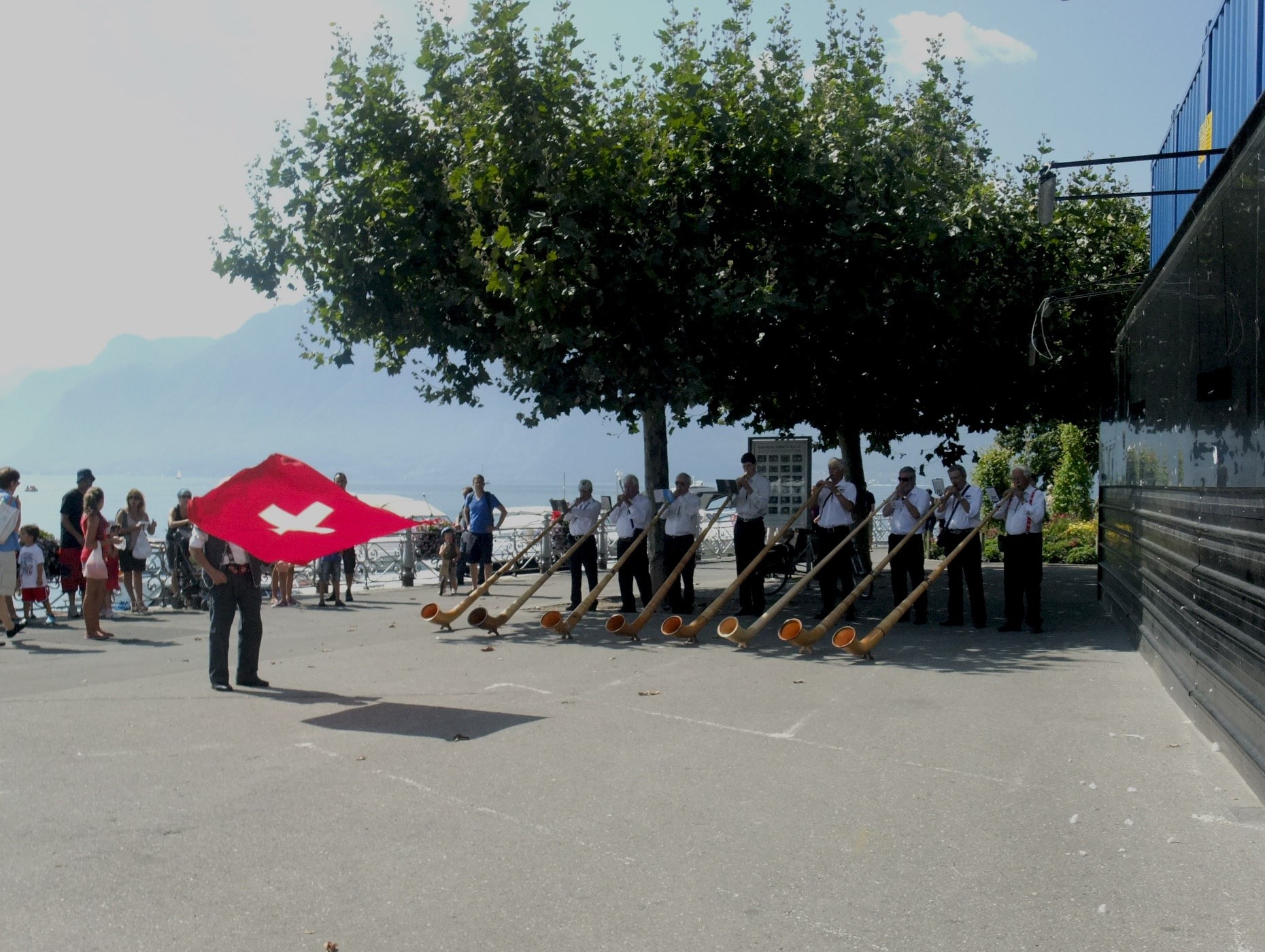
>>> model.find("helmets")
[441,526,453,537]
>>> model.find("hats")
[76,469,95,483]
[578,479,592,490]
[178,489,192,498]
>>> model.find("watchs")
[959,496,964,501]
[901,496,907,500]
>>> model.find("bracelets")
[835,491,840,496]
[1021,500,1027,504]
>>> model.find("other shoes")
[85,632,110,640]
[814,614,855,621]
[99,608,113,618]
[7,622,27,638]
[734,603,762,617]
[451,588,457,594]
[69,607,80,617]
[213,684,233,692]
[130,600,148,612]
[566,602,596,611]
[346,591,353,601]
[898,613,927,624]
[325,593,335,601]
[1000,622,1042,633]
[319,600,325,606]
[438,588,446,594]
[46,615,55,624]
[939,620,987,629]
[336,600,345,606]
[237,678,269,687]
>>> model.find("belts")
[949,529,972,534]
[572,536,580,540]
[821,526,844,533]
[737,517,752,522]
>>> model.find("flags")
[187,453,431,567]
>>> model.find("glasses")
[675,481,685,486]
[130,496,138,499]
[898,477,909,481]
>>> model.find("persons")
[883,467,930,626]
[992,467,1045,635]
[935,465,989,629]
[464,475,507,596]
[0,468,485,692]
[726,454,769,617]
[809,458,861,623]
[563,480,602,612]
[609,474,654,613]
[658,473,700,614]
[760,482,937,579]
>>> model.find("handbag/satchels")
[132,529,152,559]
[83,540,108,579]
[0,496,20,544]
[936,527,948,547]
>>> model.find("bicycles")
[762,527,874,601]
